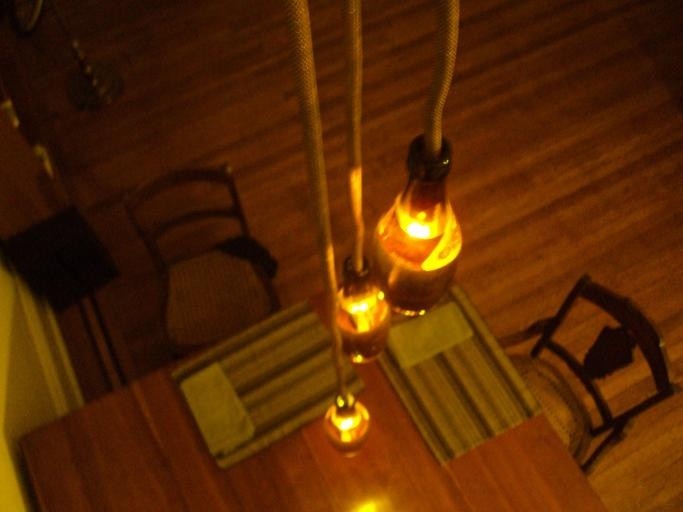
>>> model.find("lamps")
[372,0,462,319]
[290,0,372,452]
[329,0,391,369]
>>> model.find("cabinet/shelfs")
[0,77,116,313]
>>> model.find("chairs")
[489,271,682,473]
[117,166,279,356]
